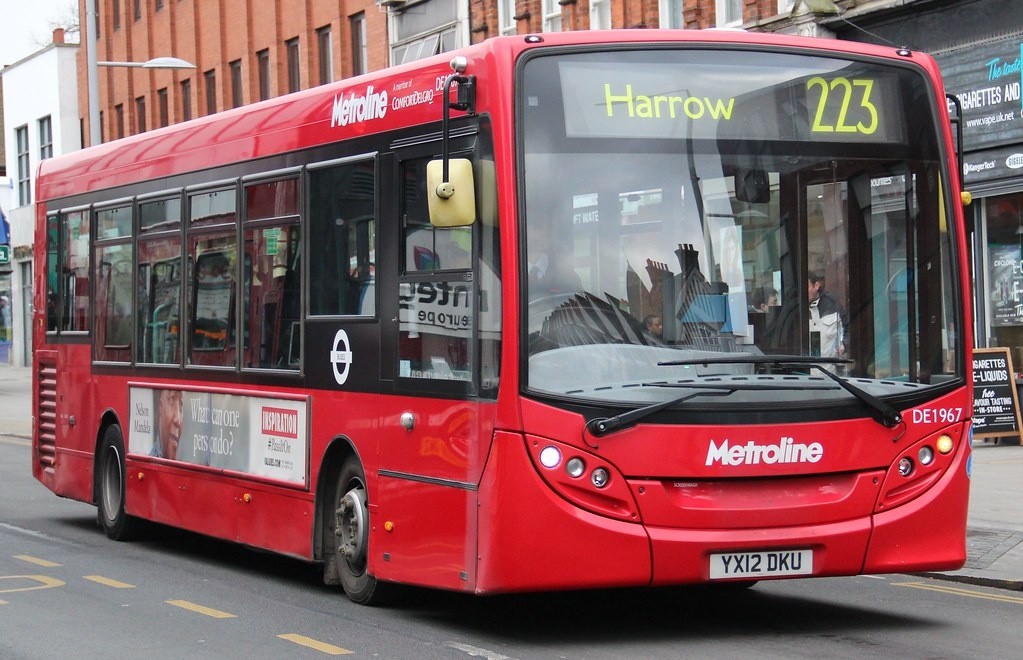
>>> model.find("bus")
[31,29,973,607]
[74,213,306,371]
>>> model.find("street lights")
[87,55,199,148]
[701,210,768,349]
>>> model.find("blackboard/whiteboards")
[987,244,1023,327]
[972,346,1023,438]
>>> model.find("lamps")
[513,12,531,22]
[558,0,577,5]
[472,24,488,33]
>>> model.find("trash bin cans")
[1000,370,1023,446]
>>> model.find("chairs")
[69,249,375,365]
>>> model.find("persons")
[164,247,249,364]
[526,223,586,315]
[749,287,779,312]
[807,268,850,379]
[641,315,663,341]
